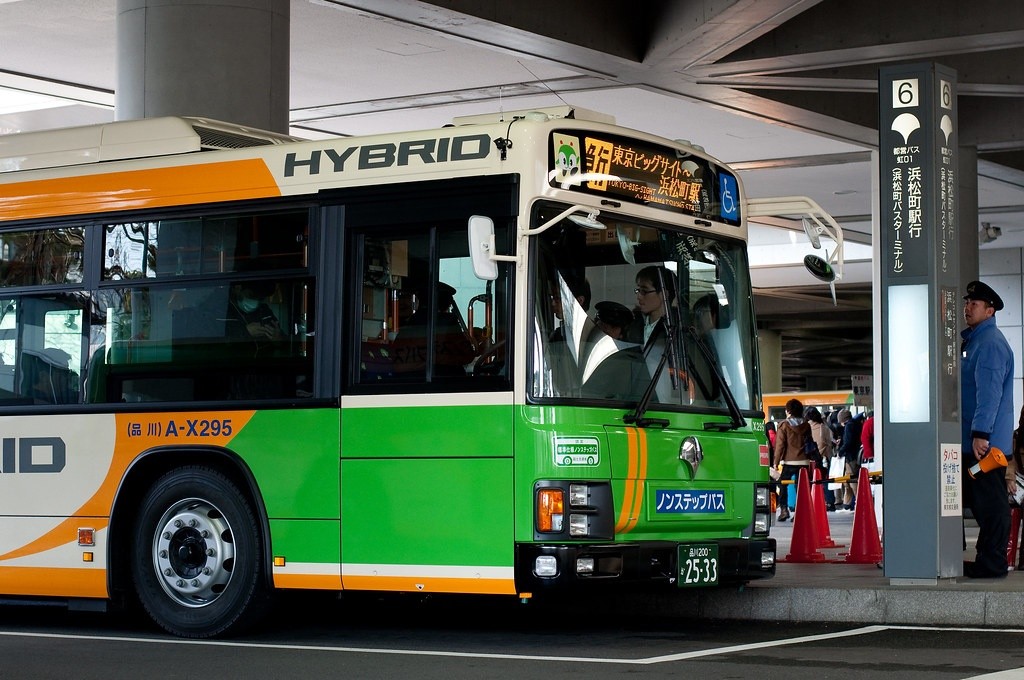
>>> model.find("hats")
[837,409,851,423]
[963,281,1004,312]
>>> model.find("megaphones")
[968,446,1008,479]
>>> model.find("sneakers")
[791,518,794,521]
[778,508,790,522]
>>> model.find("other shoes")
[964,560,1008,580]
[827,503,836,511]
[844,504,851,510]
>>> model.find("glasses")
[634,288,657,295]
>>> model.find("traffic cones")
[832,467,882,564]
[775,468,838,563]
[810,468,845,549]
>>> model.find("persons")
[961,281,1014,579]
[591,300,636,343]
[764,406,878,517]
[392,284,474,392]
[546,274,628,402]
[171,262,290,402]
[1005,404,1024,573]
[691,292,730,402]
[773,399,815,522]
[629,266,720,413]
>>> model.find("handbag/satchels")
[804,428,820,458]
[828,451,845,490]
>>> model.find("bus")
[762,390,873,424]
[0,116,843,641]
